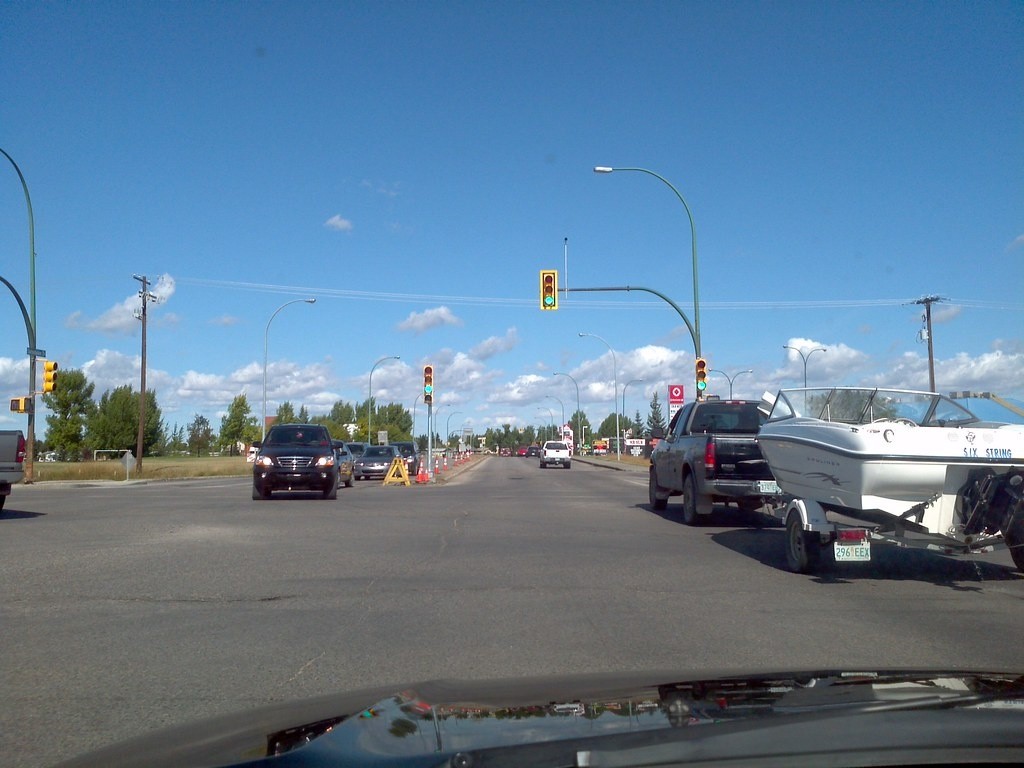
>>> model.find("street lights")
[621,430,625,443]
[260,299,316,441]
[553,372,581,456]
[434,404,463,457]
[545,395,564,441]
[783,345,827,417]
[583,426,588,445]
[595,166,700,400]
[623,379,643,449]
[369,355,400,446]
[579,333,620,460]
[538,407,553,441]
[709,368,752,401]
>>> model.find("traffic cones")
[414,460,429,483]
[433,451,469,473]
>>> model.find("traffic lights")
[9,397,31,413]
[44,361,57,393]
[423,365,433,404]
[695,358,707,392]
[540,270,558,310]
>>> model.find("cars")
[251,424,418,500]
[516,445,540,458]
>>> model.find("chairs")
[736,411,756,428]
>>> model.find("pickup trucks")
[0,429,27,512]
[649,401,776,526]
[540,440,571,469]
